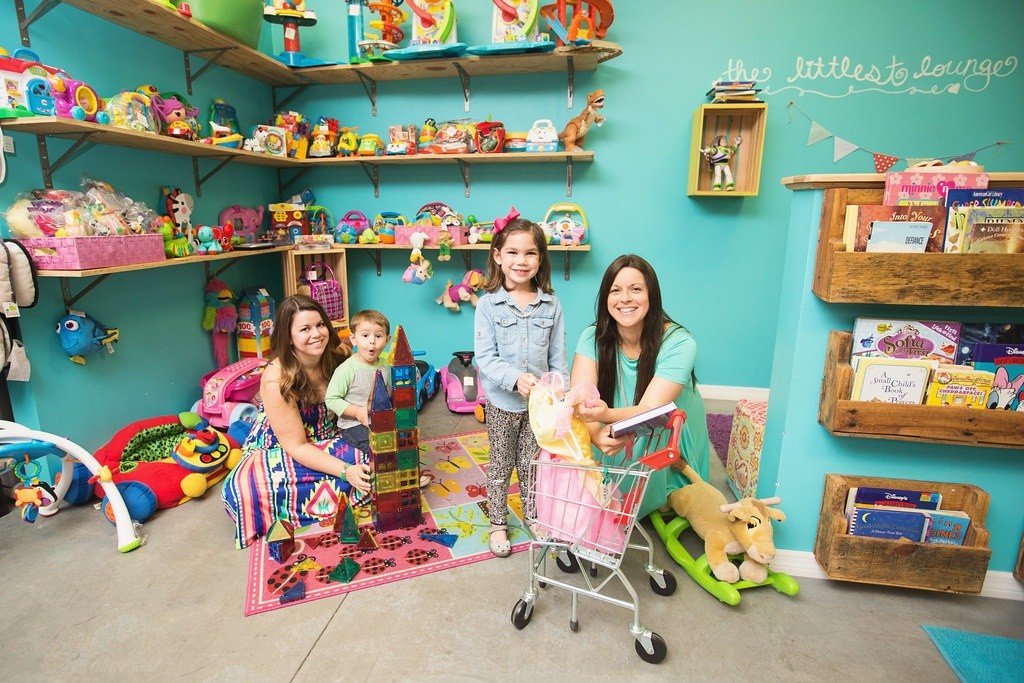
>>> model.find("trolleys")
[509,408,688,666]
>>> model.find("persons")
[699,135,743,192]
[472,208,566,557]
[221,293,371,550]
[324,310,392,455]
[569,254,709,523]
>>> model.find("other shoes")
[524,518,538,540]
[488,523,511,557]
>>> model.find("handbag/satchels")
[302,262,343,320]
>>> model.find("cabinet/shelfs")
[811,186,1024,308]
[813,473,992,596]
[0,0,599,320]
[818,329,1024,450]
[283,248,350,335]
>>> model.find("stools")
[725,399,769,504]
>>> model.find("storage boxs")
[882,171,991,209]
[10,232,167,270]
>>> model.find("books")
[608,401,676,437]
[843,167,1024,254]
[851,317,1024,411]
[705,79,764,103]
[844,485,971,547]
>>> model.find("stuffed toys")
[201,279,238,370]
[658,457,786,586]
[409,231,430,263]
[401,259,433,285]
[434,231,455,262]
[55,312,119,366]
[435,269,488,312]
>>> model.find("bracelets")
[340,463,351,483]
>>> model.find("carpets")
[243,428,563,618]
[705,412,734,469]
[920,624,1024,683]
